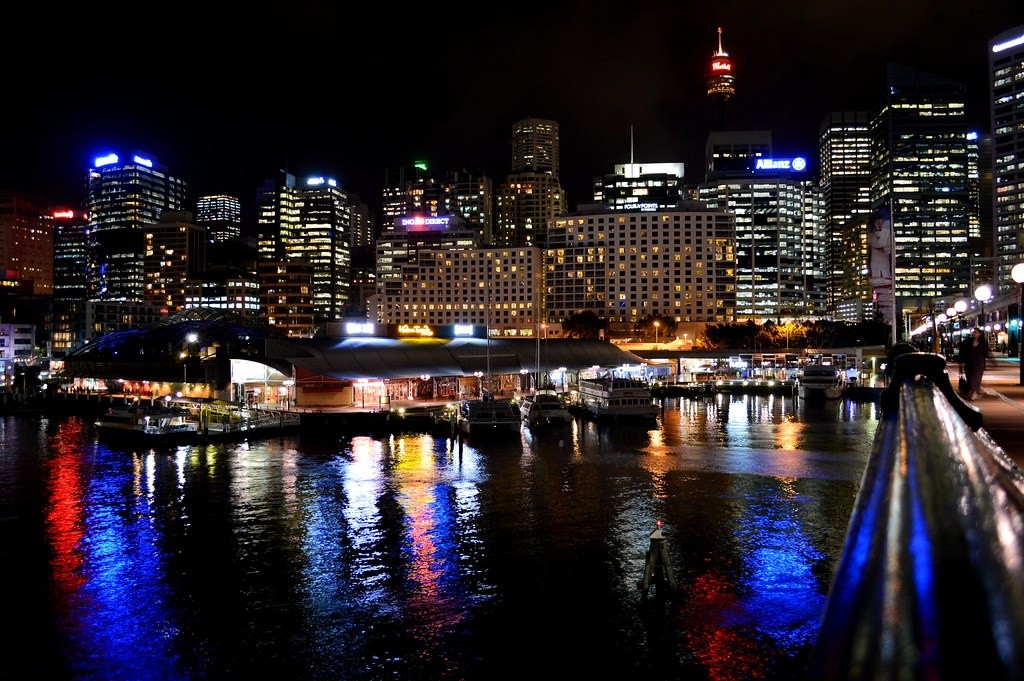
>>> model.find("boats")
[799,364,845,400]
[93,404,197,443]
[575,375,660,424]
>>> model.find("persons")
[958,327,999,402]
[905,336,1008,363]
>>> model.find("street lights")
[1010,262,1024,386]
[911,300,968,374]
[974,286,991,333]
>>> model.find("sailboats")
[459,305,520,434]
[517,281,575,429]
